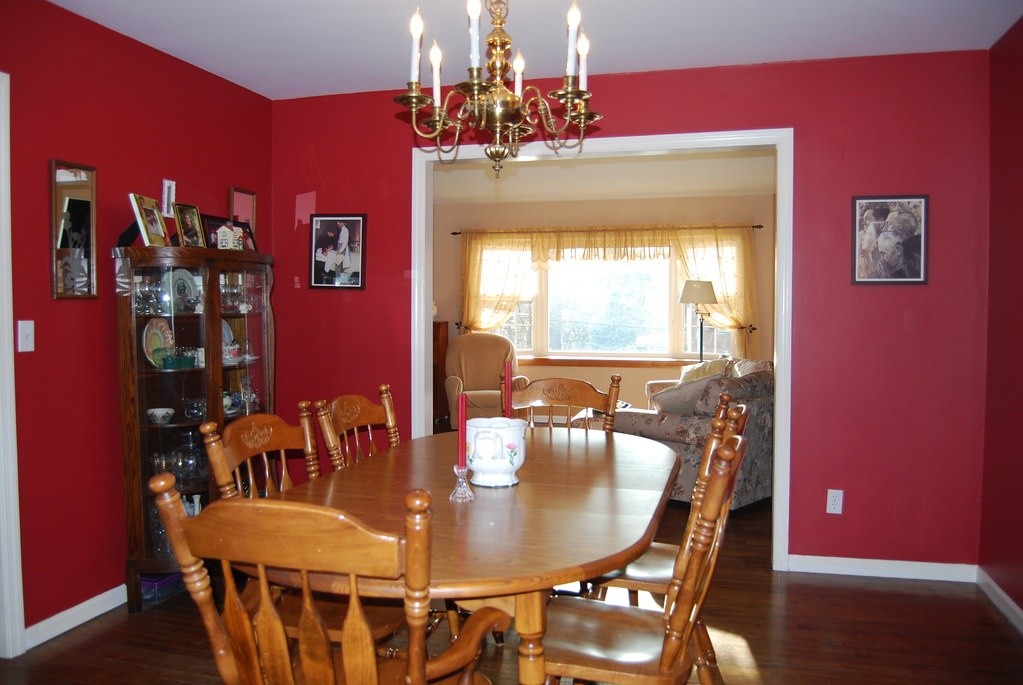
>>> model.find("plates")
[168,269,198,302]
[222,358,245,364]
[222,319,233,347]
[241,356,260,361]
[142,317,174,367]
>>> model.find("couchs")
[445,333,530,429]
[614,371,776,516]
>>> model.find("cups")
[197,348,205,367]
[222,346,238,360]
[239,304,252,314]
[166,346,196,357]
[466,417,528,488]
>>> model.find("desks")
[234,426,681,685]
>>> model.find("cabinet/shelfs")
[112,246,276,612]
[433,320,450,424]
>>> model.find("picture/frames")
[309,212,367,289]
[200,214,230,248]
[231,221,256,248]
[129,193,172,248]
[851,195,928,284]
[172,202,207,248]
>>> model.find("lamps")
[678,280,718,362]
[392,0,603,178]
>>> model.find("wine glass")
[151,451,196,491]
[221,285,242,313]
[135,281,167,316]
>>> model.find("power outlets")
[826,489,843,514]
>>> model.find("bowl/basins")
[147,408,175,424]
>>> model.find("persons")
[857,207,921,279]
[183,212,199,245]
[314,221,351,284]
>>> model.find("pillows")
[651,374,723,415]
[679,358,728,384]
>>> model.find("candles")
[505,360,512,417]
[458,393,467,469]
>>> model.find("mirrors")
[50,159,98,300]
[229,185,257,288]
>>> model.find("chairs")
[499,371,620,432]
[148,383,511,684]
[542,394,752,685]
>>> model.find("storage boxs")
[140,573,185,606]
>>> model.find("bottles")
[185,400,207,420]
